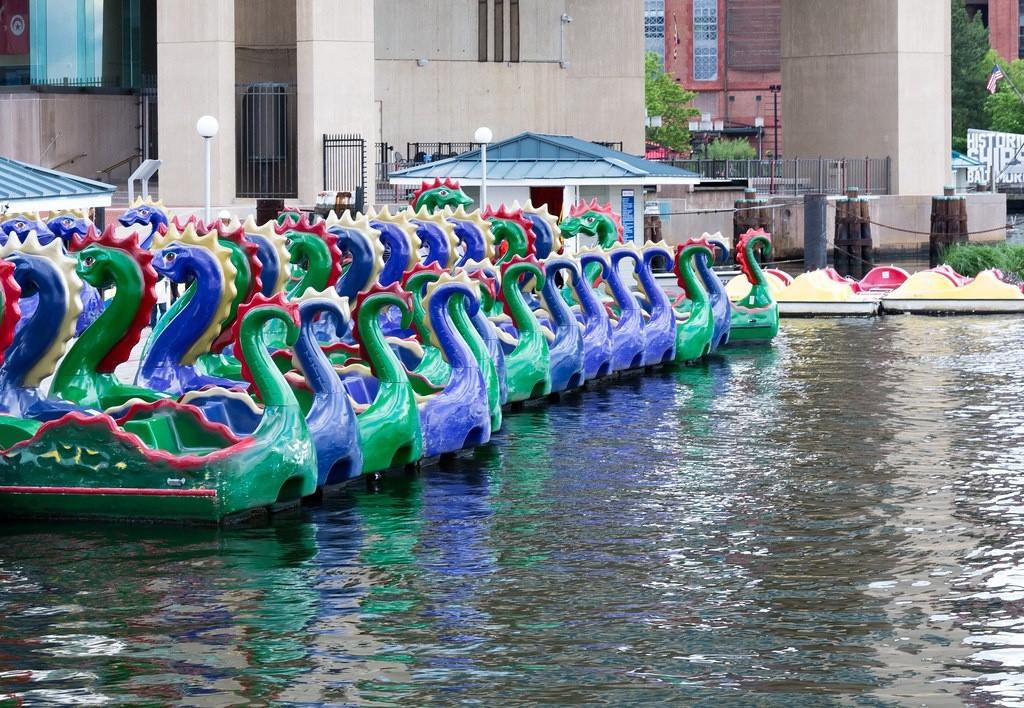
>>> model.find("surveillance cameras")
[566,18,572,23]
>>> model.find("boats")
[763,266,911,316]
[882,268,1024,315]
[0,179,777,520]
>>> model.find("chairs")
[414,152,458,162]
[395,151,410,171]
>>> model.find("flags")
[986,63,1003,94]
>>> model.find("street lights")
[769,83,781,159]
